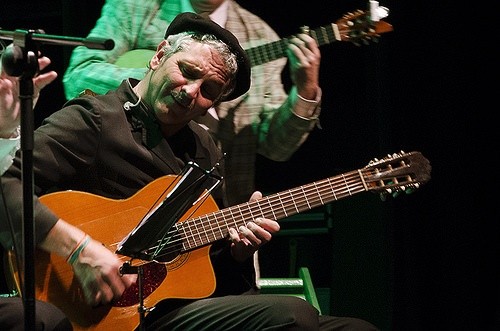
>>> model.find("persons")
[62,0,323,207]
[0,42,74,331]
[0,12,379,331]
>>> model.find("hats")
[165,12,251,102]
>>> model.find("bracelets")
[65,231,90,266]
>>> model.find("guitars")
[8,150,433,331]
[115,9,394,70]
